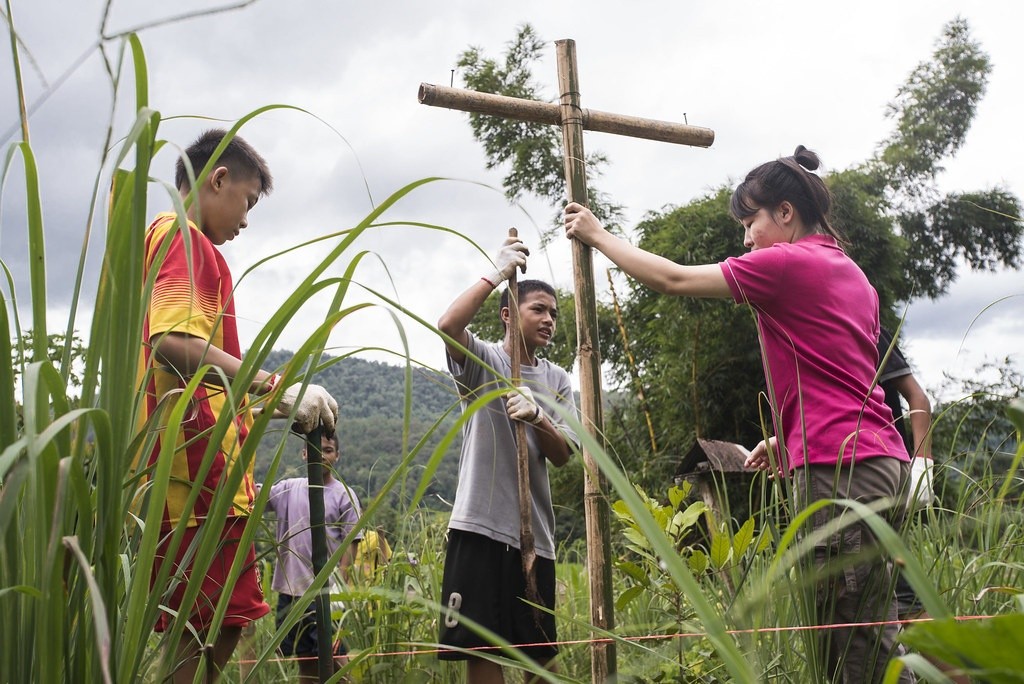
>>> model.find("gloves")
[267,373,339,440]
[500,386,543,424]
[482,236,530,288]
[903,455,934,514]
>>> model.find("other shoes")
[334,638,353,670]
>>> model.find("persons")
[438,237,581,684]
[256,426,392,684]
[564,145,934,684]
[137,128,338,684]
